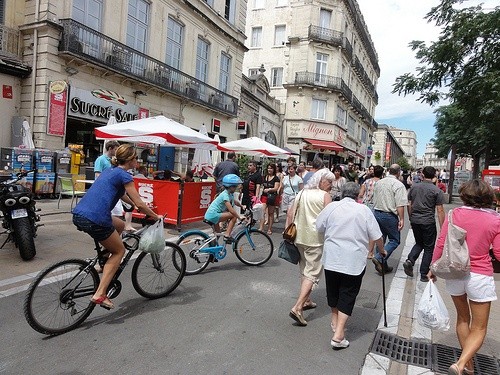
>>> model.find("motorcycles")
[0,168,45,261]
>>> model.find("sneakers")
[420,275,429,282]
[403,260,414,277]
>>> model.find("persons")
[94,141,136,232]
[403,166,445,282]
[239,161,264,230]
[280,165,304,235]
[316,182,387,349]
[134,166,218,182]
[70,144,165,308]
[368,164,409,275]
[357,166,384,258]
[284,168,336,326]
[257,163,280,235]
[270,162,284,223]
[204,173,247,262]
[287,155,453,203]
[213,152,242,230]
[426,178,500,375]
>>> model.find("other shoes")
[264,221,269,225]
[373,257,393,275]
[223,235,239,242]
[273,218,279,223]
[258,229,272,235]
[209,257,218,262]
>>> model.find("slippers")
[90,296,115,309]
[289,310,307,326]
[331,321,337,333]
[303,300,317,311]
[331,337,350,348]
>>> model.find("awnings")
[304,139,344,156]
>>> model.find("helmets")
[222,174,244,188]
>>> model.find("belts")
[375,209,394,215]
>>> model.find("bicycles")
[23,209,187,337]
[173,207,274,277]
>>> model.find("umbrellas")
[191,122,212,178]
[217,136,291,161]
[22,120,35,149]
[93,115,218,164]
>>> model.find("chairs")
[57,176,88,212]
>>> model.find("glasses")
[135,156,138,160]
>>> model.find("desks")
[77,180,96,184]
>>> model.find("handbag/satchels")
[417,279,451,332]
[278,240,301,265]
[267,193,282,206]
[431,209,470,280]
[283,223,296,241]
[139,217,166,254]
